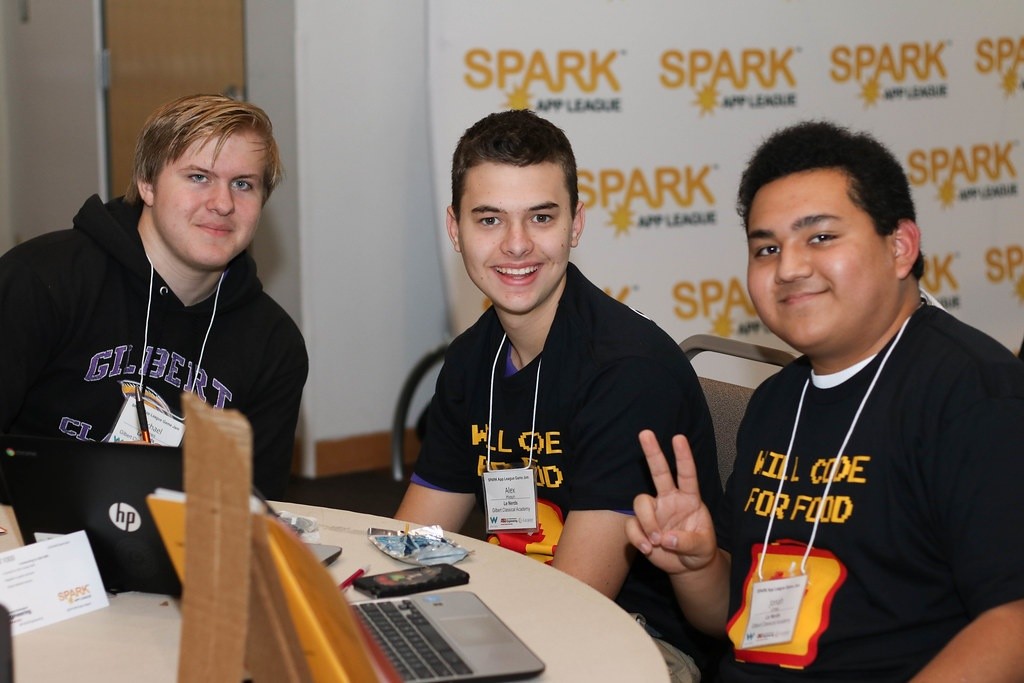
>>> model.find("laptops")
[253,484,546,683]
[0,434,343,599]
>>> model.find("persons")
[390,108,724,682]
[622,121,1024,683]
[0,91,311,500]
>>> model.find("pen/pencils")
[134,385,151,443]
[336,562,373,591]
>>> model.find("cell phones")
[352,563,470,599]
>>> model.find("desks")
[1,501,672,683]
